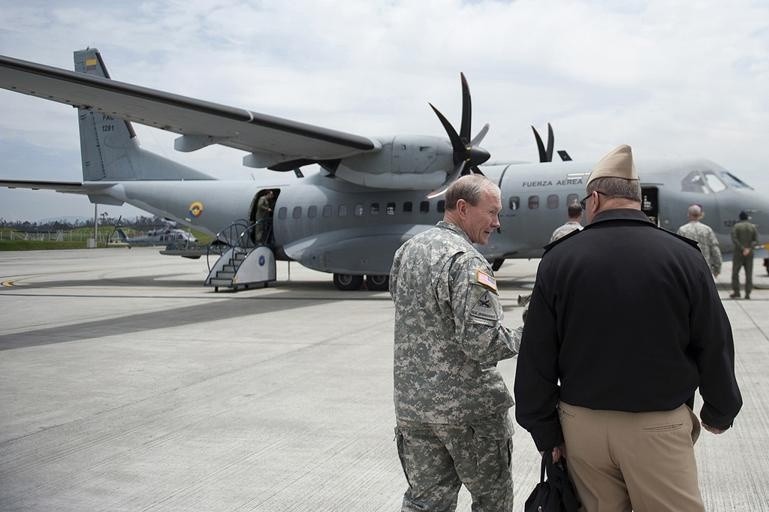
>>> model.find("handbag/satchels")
[524,448,579,512]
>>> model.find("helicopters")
[110,214,198,251]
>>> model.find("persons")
[513,142,744,512]
[548,203,585,246]
[677,204,722,282]
[255,189,274,246]
[388,172,526,512]
[729,211,759,300]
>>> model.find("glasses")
[579,190,608,210]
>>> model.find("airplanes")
[0,49,769,294]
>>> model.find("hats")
[569,198,582,210]
[585,144,640,190]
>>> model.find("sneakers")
[729,292,751,299]
